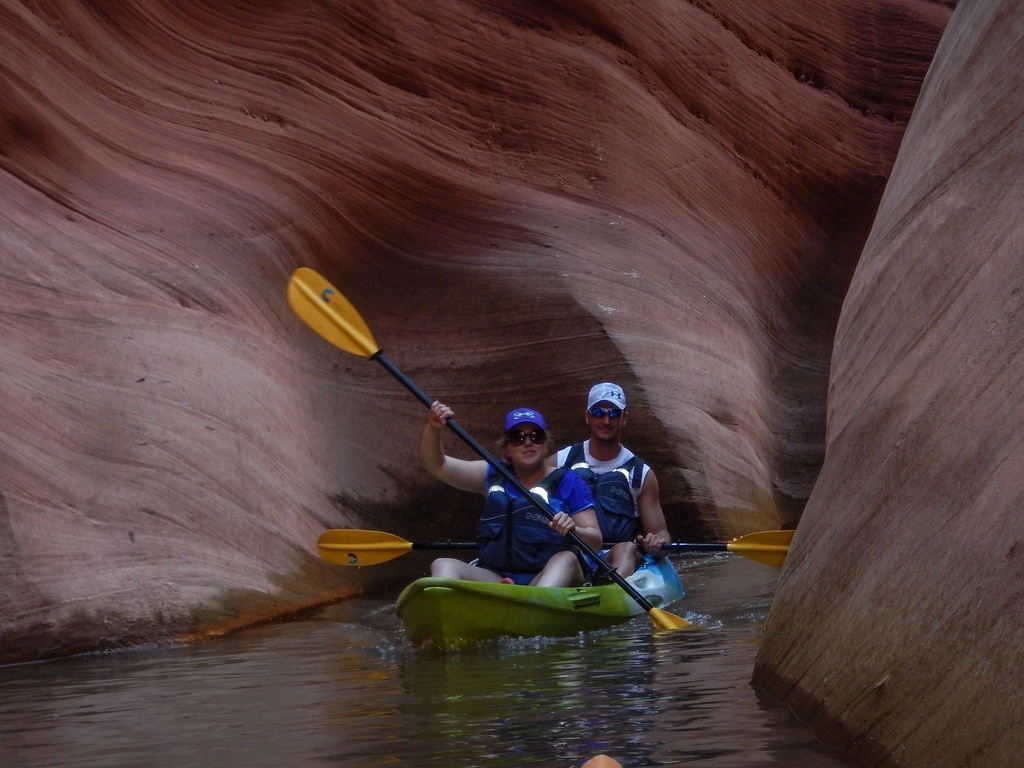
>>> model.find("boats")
[394,550,685,653]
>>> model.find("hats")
[502,408,548,433]
[586,383,626,411]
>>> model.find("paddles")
[315,526,797,569]
[282,264,693,638]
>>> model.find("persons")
[421,400,603,586]
[545,383,672,581]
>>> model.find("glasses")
[589,406,623,418]
[501,426,547,446]
